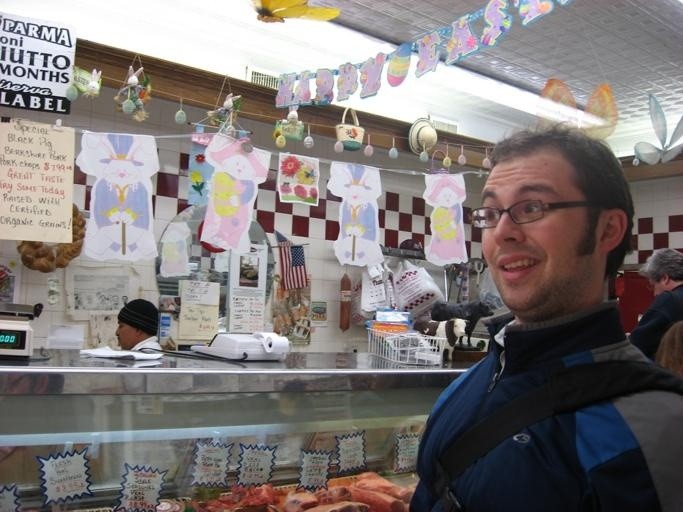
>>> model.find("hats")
[115,298,160,338]
[409,117,439,156]
[343,164,372,190]
[427,177,465,203]
[100,133,146,168]
[207,139,267,179]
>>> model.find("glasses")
[471,195,603,229]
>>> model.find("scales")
[0,303,43,361]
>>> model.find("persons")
[629,248,683,359]
[116,299,162,351]
[655,320,683,378]
[410,118,683,512]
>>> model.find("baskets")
[367,327,449,367]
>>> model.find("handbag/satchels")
[335,105,366,153]
